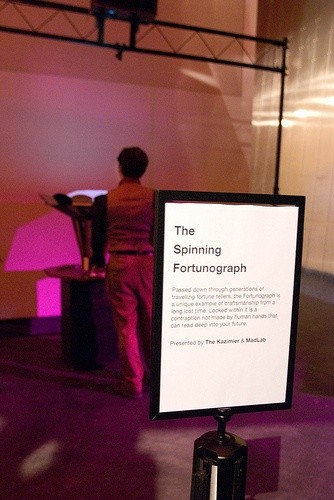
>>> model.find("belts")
[116,250,151,256]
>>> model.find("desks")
[43,264,118,364]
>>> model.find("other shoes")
[113,381,143,398]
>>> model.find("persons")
[88,144,156,400]
